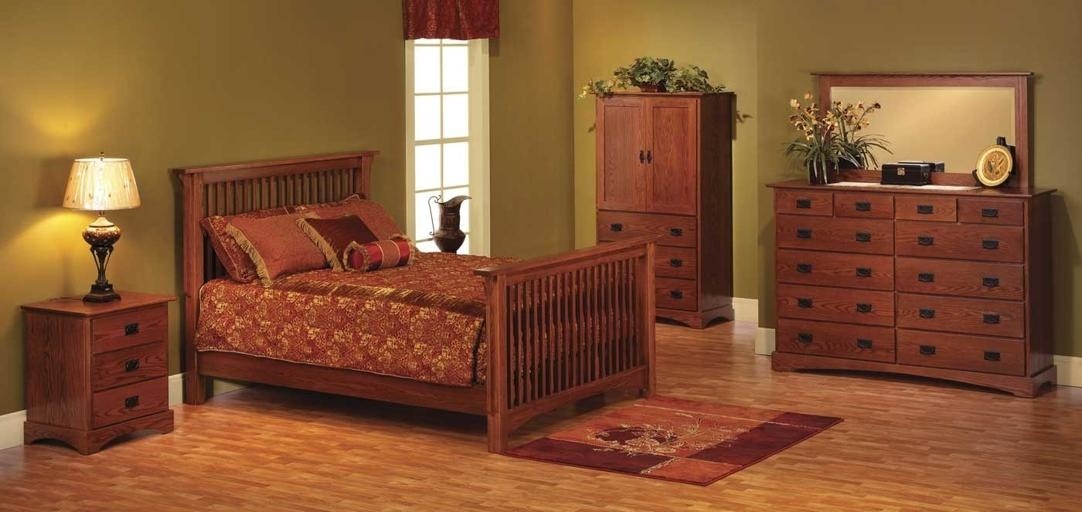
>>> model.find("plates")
[975,144,1013,189]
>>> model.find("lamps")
[62,157,142,303]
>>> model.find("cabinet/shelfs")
[594,91,734,329]
[767,183,1056,397]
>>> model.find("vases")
[806,163,837,185]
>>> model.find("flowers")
[783,93,847,163]
[831,98,893,169]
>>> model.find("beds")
[179,151,656,454]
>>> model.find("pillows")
[226,213,327,288]
[344,235,414,272]
[201,208,296,282]
[296,213,379,272]
[302,199,404,241]
[296,193,366,213]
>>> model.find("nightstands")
[21,290,177,455]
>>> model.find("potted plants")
[570,58,728,97]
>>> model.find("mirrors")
[811,72,1032,175]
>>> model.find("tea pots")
[427,194,473,253]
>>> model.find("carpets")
[503,395,844,486]
